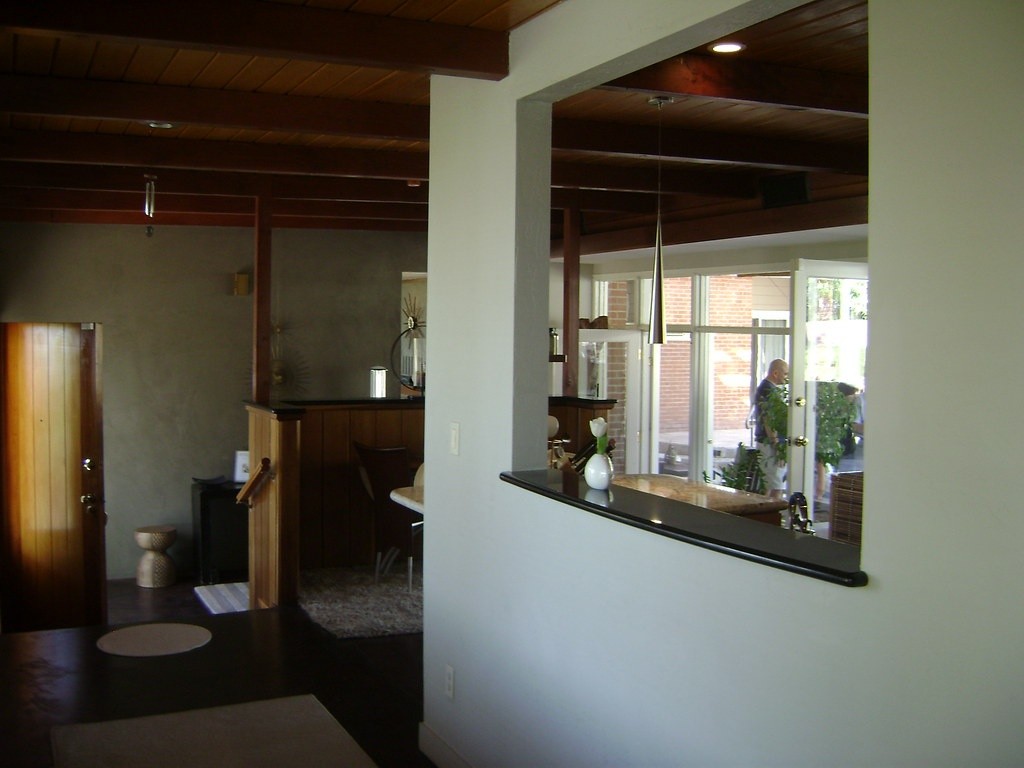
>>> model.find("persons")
[754,358,789,503]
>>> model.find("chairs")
[352,440,423,593]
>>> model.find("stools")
[135,526,178,590]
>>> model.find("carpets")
[298,560,424,641]
